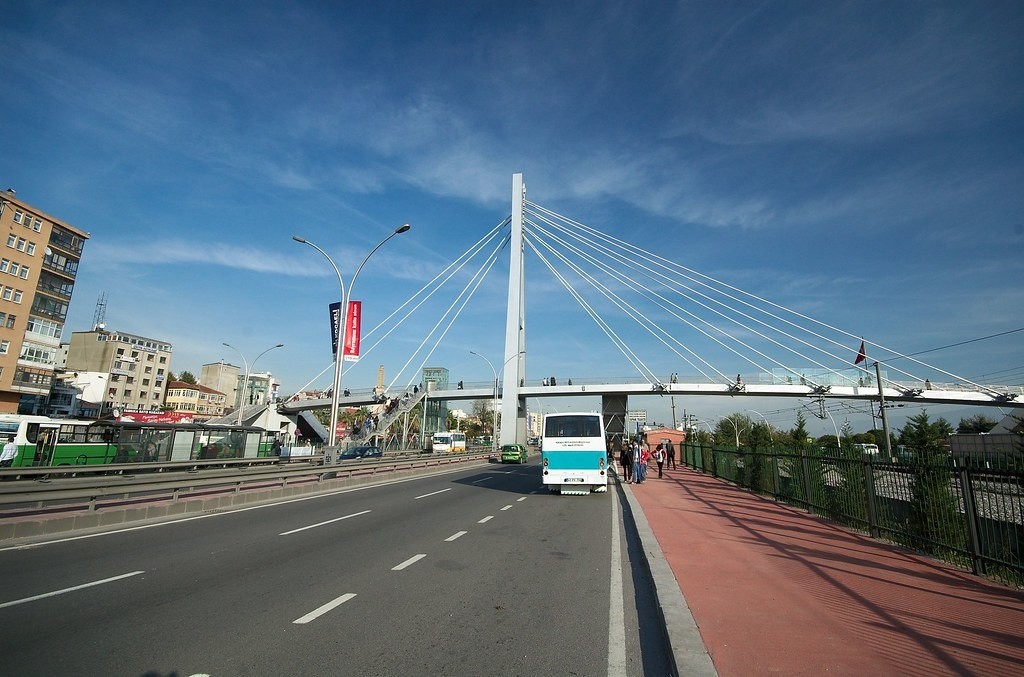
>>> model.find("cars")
[501,443,529,464]
[338,446,383,460]
[897,444,916,458]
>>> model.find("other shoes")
[624,480,645,484]
[674,467,677,470]
[659,475,662,478]
[668,466,670,470]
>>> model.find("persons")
[270,438,312,464]
[568,379,573,385]
[800,374,807,385]
[666,439,676,470]
[925,378,931,390]
[275,393,299,403]
[343,388,351,397]
[619,439,649,484]
[543,377,556,386]
[249,393,253,404]
[458,380,463,390]
[385,397,399,417]
[204,442,230,469]
[105,443,156,476]
[414,385,419,396]
[651,445,666,478]
[365,412,379,430]
[858,376,873,387]
[607,442,615,461]
[353,424,360,441]
[669,372,678,384]
[327,389,333,398]
[419,383,422,392]
[0,437,18,468]
[737,373,742,384]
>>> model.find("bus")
[0,414,161,477]
[541,412,608,494]
[431,432,466,455]
[853,443,880,455]
[191,424,281,460]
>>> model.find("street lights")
[718,415,746,450]
[748,410,781,448]
[222,342,284,425]
[801,399,848,448]
[293,223,411,447]
[470,351,527,452]
[697,418,714,438]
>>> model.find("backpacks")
[655,449,663,462]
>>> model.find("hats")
[629,444,633,448]
